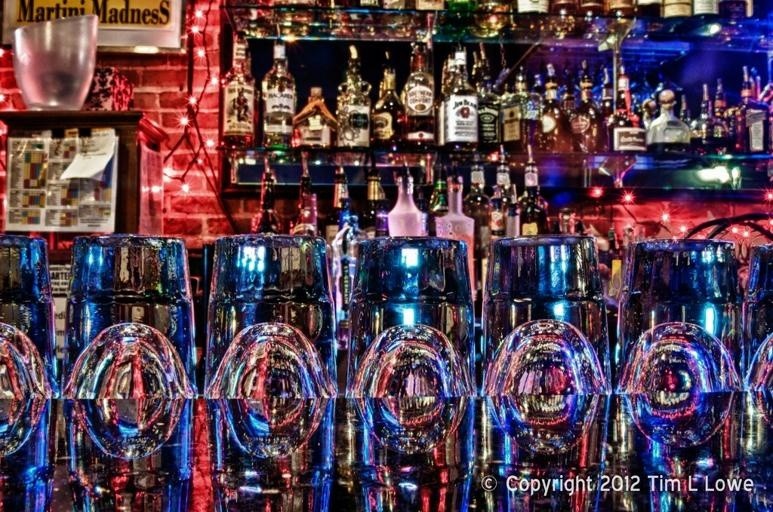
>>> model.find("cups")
[12,14,100,112]
[0,230,773,512]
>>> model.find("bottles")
[245,141,577,309]
[223,0,773,154]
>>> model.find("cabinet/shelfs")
[219,0,773,199]
[0,110,170,265]
